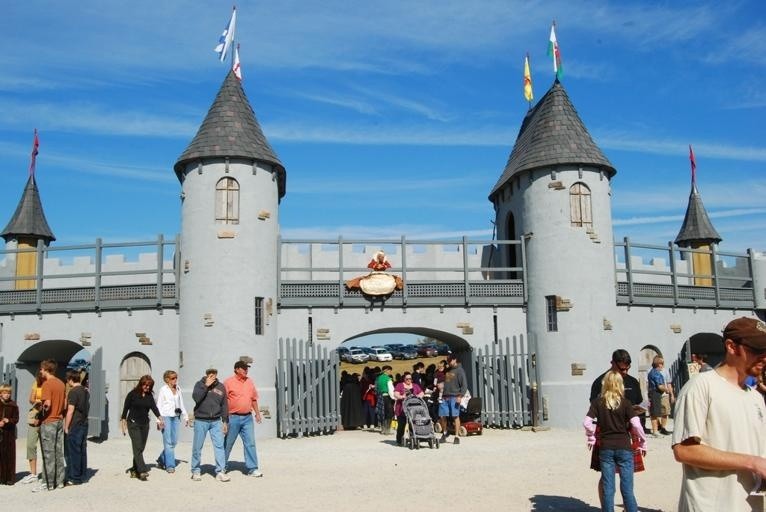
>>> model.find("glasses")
[615,361,631,372]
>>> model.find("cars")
[335,342,453,363]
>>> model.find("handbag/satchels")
[652,391,670,418]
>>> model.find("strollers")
[401,394,440,449]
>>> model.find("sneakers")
[454,437,459,443]
[652,431,664,438]
[21,473,38,484]
[32,473,88,492]
[130,469,148,479]
[192,473,201,480]
[216,471,231,482]
[248,468,263,477]
[439,431,450,443]
[156,458,175,473]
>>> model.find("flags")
[524,56,533,102]
[547,24,564,84]
[28,132,39,176]
[232,46,242,81]
[214,9,237,62]
[688,145,696,185]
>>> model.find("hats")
[205,363,217,374]
[234,361,250,368]
[724,316,766,351]
[446,352,456,362]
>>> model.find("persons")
[339,354,472,445]
[157,371,189,473]
[120,375,165,480]
[368,252,392,271]
[191,367,231,482]
[223,361,264,477]
[0,384,19,485]
[583,317,766,512]
[22,359,90,493]
